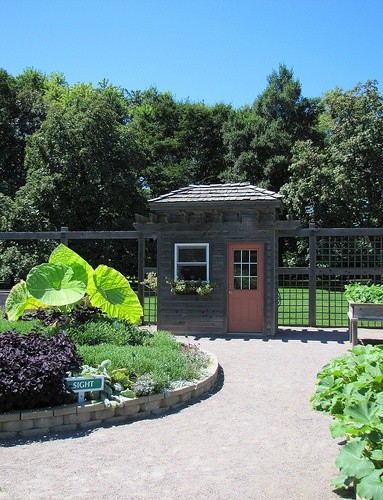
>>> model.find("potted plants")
[344,281,383,346]
